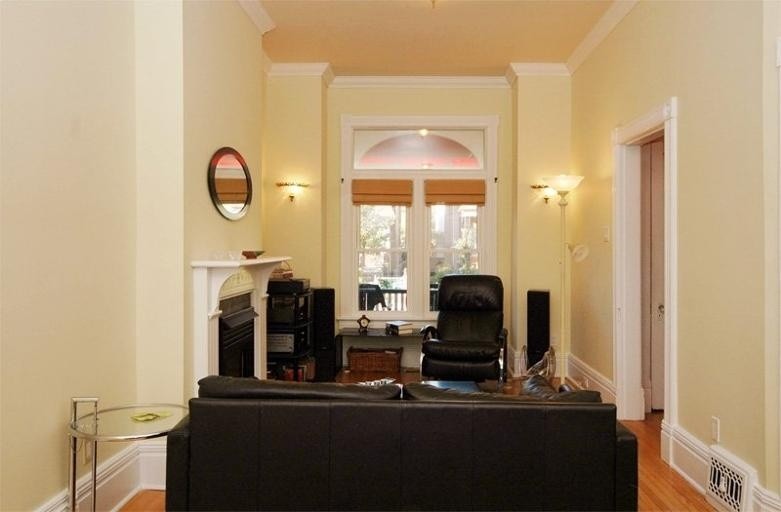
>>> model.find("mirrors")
[208,146,255,222]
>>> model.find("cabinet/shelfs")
[265,291,315,383]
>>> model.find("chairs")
[359,283,391,310]
[417,274,509,382]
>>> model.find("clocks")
[356,315,372,334]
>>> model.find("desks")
[334,326,428,372]
[384,379,481,399]
[63,392,190,511]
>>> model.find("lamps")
[274,179,311,202]
[531,185,560,203]
[542,172,588,386]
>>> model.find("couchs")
[165,373,641,512]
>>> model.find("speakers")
[527,290,550,371]
[312,289,335,379]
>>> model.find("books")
[384,320,413,335]
[267,358,317,382]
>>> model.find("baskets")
[347,346,402,373]
